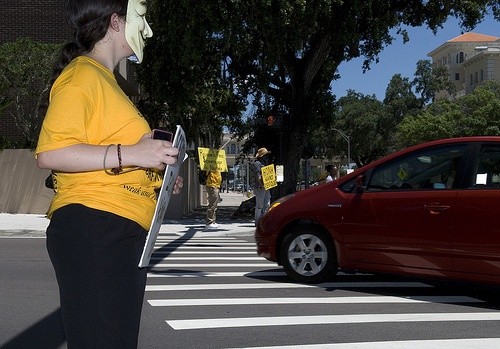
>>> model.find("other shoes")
[206,223,221,230]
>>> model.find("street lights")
[332,128,350,171]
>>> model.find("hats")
[256,147,271,157]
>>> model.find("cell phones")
[151,128,174,143]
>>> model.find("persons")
[205,171,222,229]
[34,0,180,349]
[325,165,338,183]
[251,148,271,227]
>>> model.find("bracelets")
[117,144,123,170]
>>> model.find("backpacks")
[198,165,210,185]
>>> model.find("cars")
[228,184,245,193]
[254,135,500,286]
